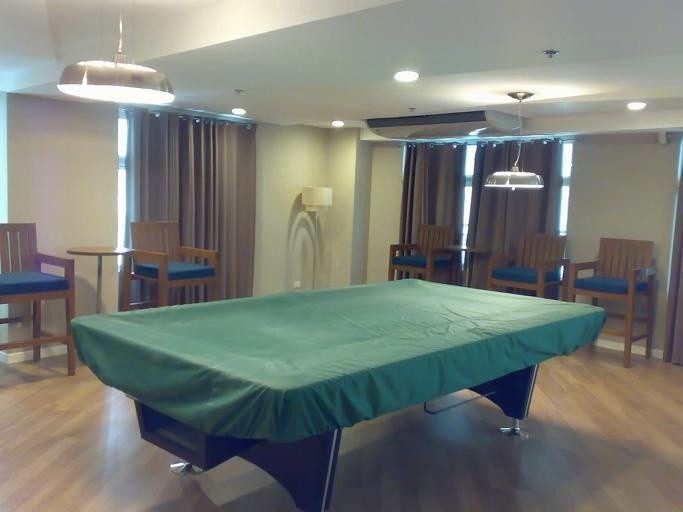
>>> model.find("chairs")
[387,224,460,284]
[486,231,569,299]
[124,220,219,311]
[566,237,658,368]
[0,222,77,377]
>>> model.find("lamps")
[56,0,176,106]
[300,185,332,289]
[483,91,545,189]
[363,112,528,139]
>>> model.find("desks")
[66,245,134,314]
[447,243,492,286]
[71,278,608,512]
